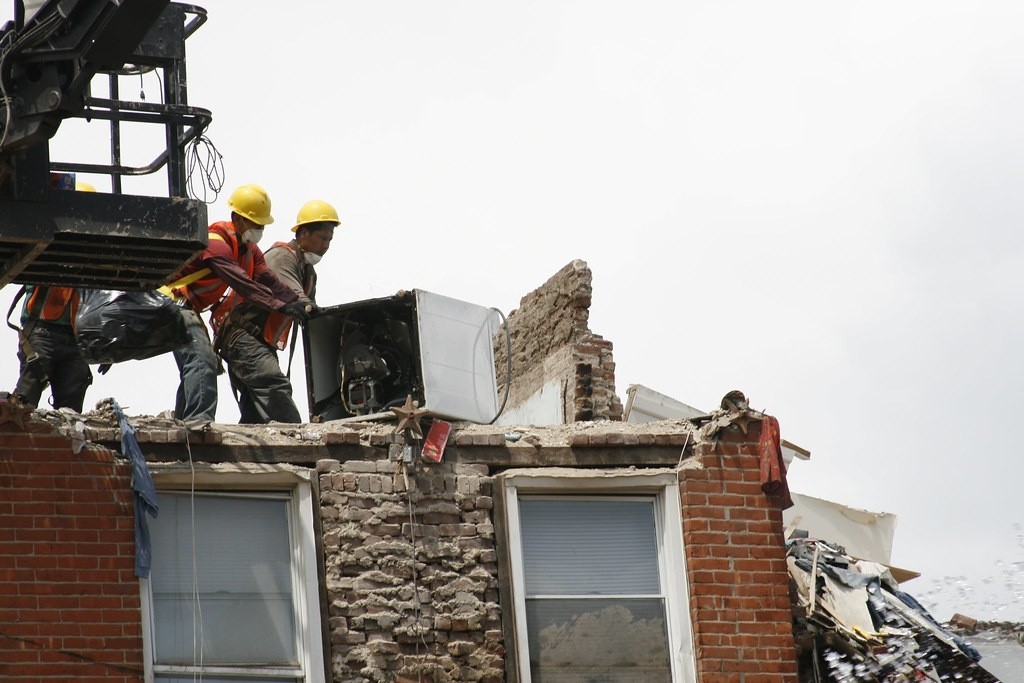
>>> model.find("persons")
[12,181,113,413]
[153,185,341,424]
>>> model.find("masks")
[234,217,263,243]
[302,251,323,266]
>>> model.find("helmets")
[227,184,274,224]
[290,199,341,232]
